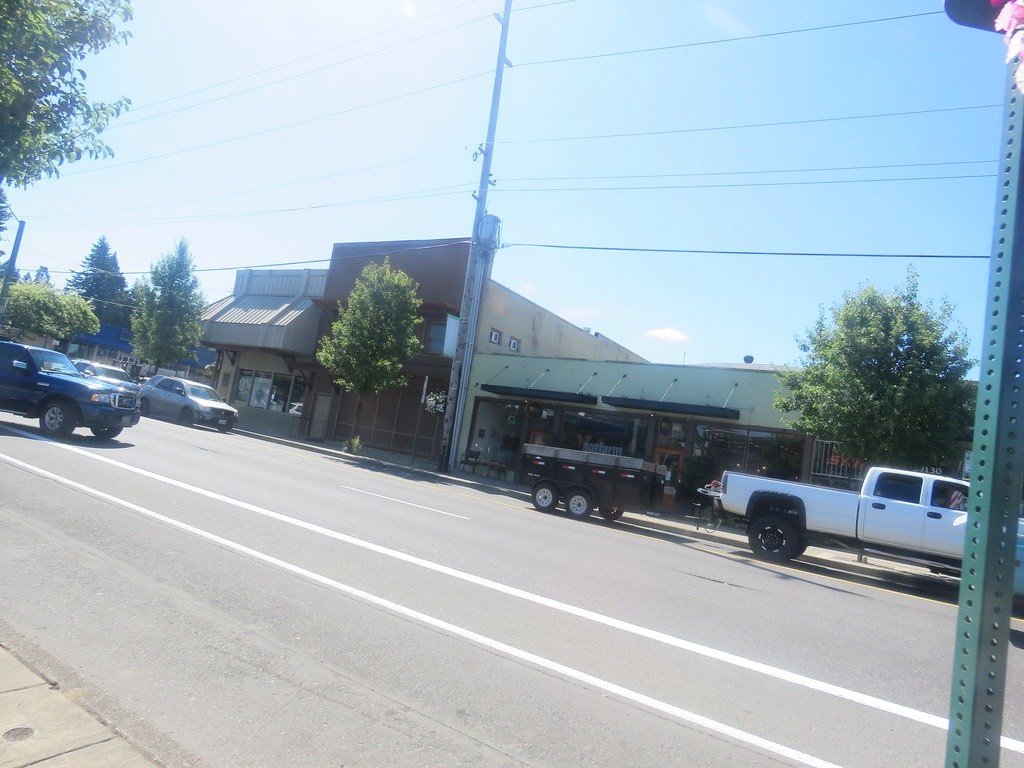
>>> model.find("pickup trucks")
[719,466,1024,592]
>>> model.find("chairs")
[458,450,481,474]
[486,456,512,482]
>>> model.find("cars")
[288,402,303,415]
[0,339,143,441]
[73,354,147,396]
[136,372,241,434]
